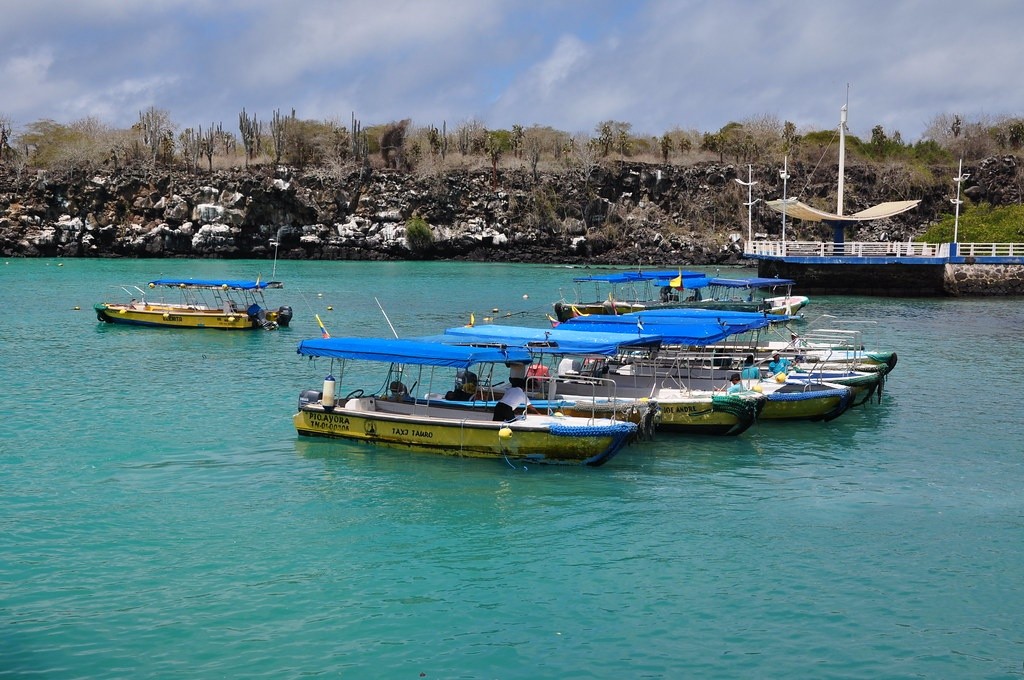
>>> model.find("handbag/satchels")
[602,363,609,373]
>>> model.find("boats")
[92,278,293,330]
[291,254,899,470]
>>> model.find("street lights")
[950,158,972,243]
[736,163,759,254]
[778,154,791,257]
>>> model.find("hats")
[730,374,740,381]
[791,333,798,336]
[772,351,779,358]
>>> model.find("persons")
[727,373,746,395]
[685,288,702,301]
[492,380,541,423]
[769,351,794,375]
[505,363,525,387]
[247,303,262,328]
[659,287,674,302]
[446,370,478,401]
[742,355,764,381]
[791,332,804,362]
